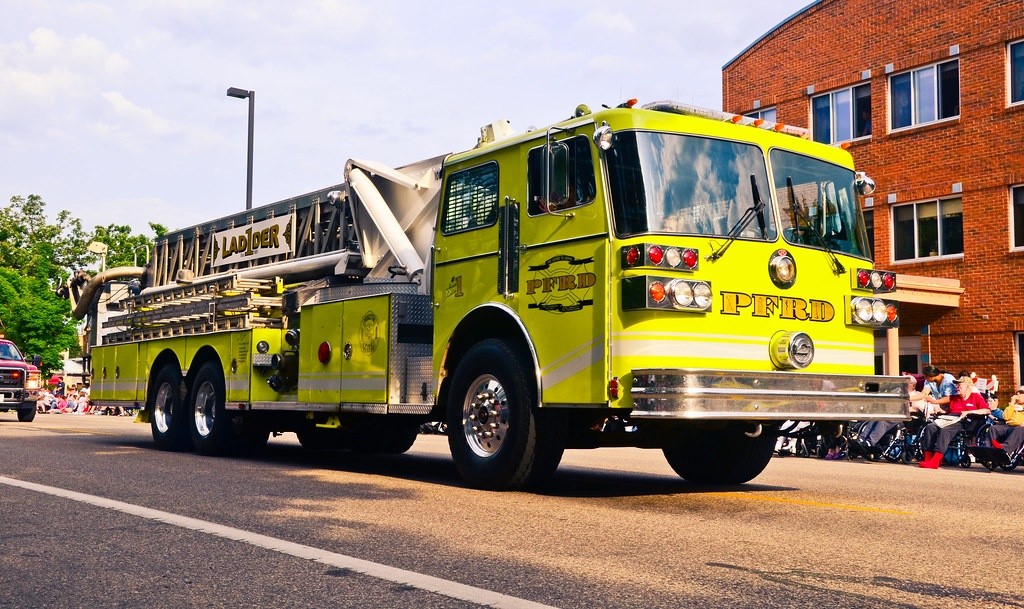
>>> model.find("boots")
[926,451,943,469]
[919,453,932,468]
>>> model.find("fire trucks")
[90,101,916,491]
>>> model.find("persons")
[33,376,133,416]
[771,366,1024,469]
[0,346,12,359]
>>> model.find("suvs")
[0,338,42,422]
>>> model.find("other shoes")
[992,439,1002,448]
[826,447,841,460]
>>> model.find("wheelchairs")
[777,413,1024,470]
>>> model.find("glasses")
[1016,390,1024,395]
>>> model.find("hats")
[952,376,974,386]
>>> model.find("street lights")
[227,87,255,210]
